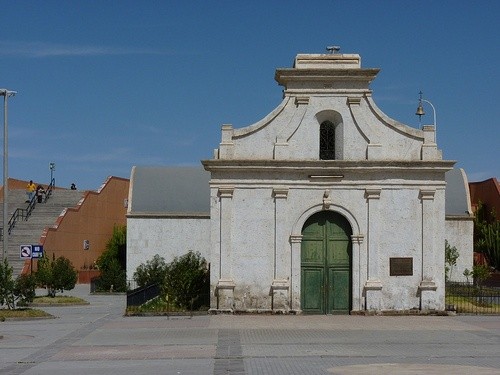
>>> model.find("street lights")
[0,88,17,282]
[49,162,55,189]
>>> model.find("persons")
[26,180,36,206]
